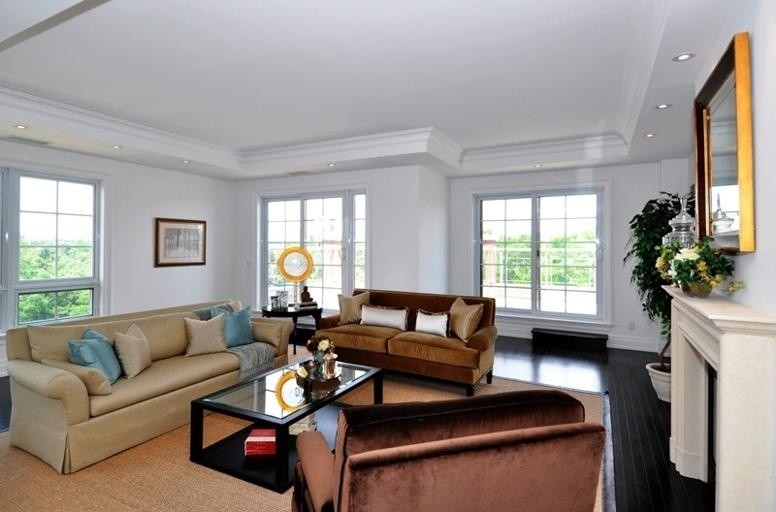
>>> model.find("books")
[288,413,317,435]
[244,427,279,456]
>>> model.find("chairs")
[288,385,608,512]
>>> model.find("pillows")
[359,303,412,333]
[111,321,154,380]
[249,321,283,348]
[446,295,486,345]
[65,328,122,385]
[337,289,371,325]
[210,305,255,350]
[181,313,230,357]
[413,307,453,339]
[38,357,114,399]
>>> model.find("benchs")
[529,327,610,367]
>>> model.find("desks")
[261,302,323,356]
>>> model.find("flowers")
[652,235,745,297]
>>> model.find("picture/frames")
[152,216,208,269]
[692,30,754,256]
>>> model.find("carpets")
[0,375,617,511]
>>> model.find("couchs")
[311,286,502,398]
[3,296,298,476]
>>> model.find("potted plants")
[624,183,696,405]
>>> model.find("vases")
[686,279,711,297]
